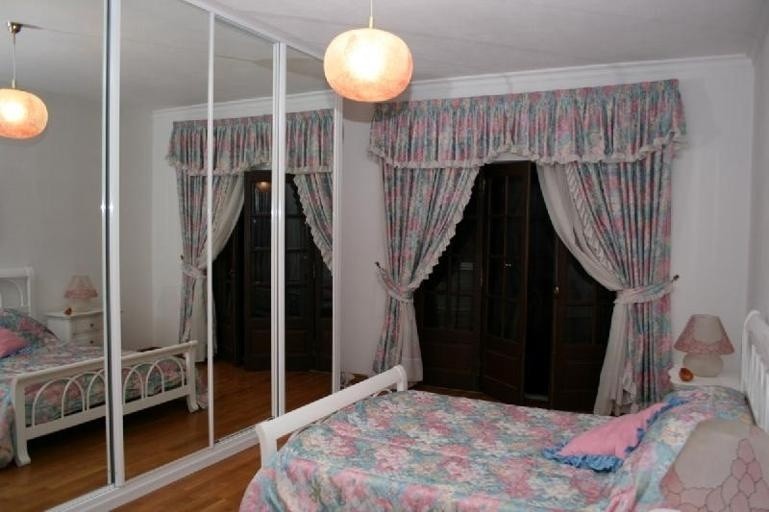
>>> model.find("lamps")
[673,314,734,377]
[63,273,98,312]
[0,22,48,140]
[659,418,769,512]
[323,0,414,102]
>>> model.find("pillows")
[540,385,754,512]
[0,307,59,359]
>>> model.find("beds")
[237,310,769,512]
[0,266,209,468]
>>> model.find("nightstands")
[668,364,741,391]
[44,308,122,347]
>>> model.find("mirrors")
[280,29,344,417]
[213,3,280,465]
[112,0,212,512]
[0,0,112,512]
